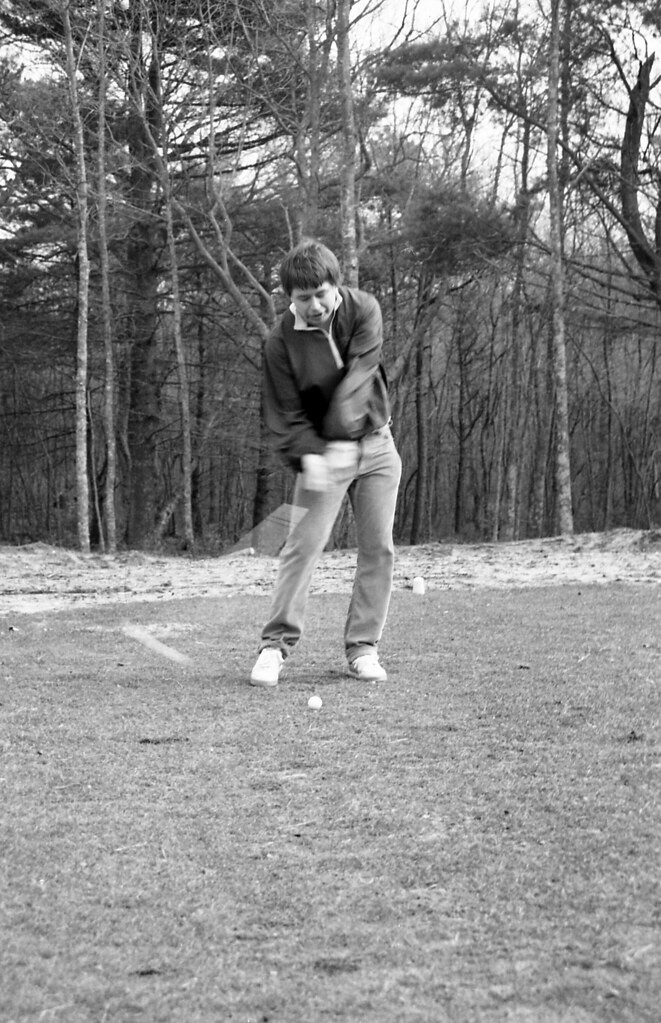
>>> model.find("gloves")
[301,454,330,492]
[326,440,362,483]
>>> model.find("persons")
[248,240,403,688]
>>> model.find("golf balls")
[308,695,323,709]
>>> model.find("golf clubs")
[125,491,320,667]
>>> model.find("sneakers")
[249,646,284,685]
[346,654,387,682]
